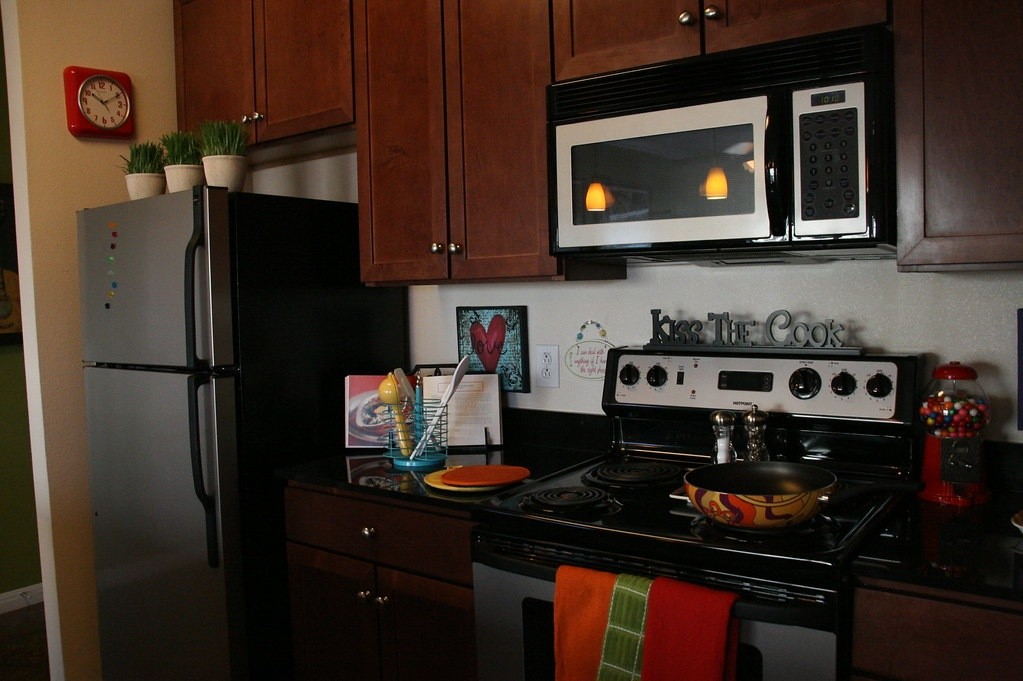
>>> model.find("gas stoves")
[477,341,929,585]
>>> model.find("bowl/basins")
[1011,510,1023,532]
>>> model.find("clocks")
[80,78,128,127]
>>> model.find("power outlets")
[535,343,561,389]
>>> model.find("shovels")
[410,354,471,460]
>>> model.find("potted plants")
[158,128,206,193]
[194,118,252,193]
[114,140,168,201]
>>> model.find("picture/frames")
[455,305,532,395]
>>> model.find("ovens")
[471,539,851,681]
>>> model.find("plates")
[424,465,531,491]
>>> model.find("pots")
[684,460,836,531]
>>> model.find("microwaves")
[546,27,897,271]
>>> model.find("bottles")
[923,361,990,508]
[742,403,770,461]
[711,410,738,464]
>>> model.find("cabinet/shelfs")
[283,481,481,681]
[172,0,357,176]
[551,0,890,87]
[890,0,1023,275]
[849,576,1023,681]
[351,0,627,288]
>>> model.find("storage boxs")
[419,373,506,447]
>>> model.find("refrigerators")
[75,186,409,681]
